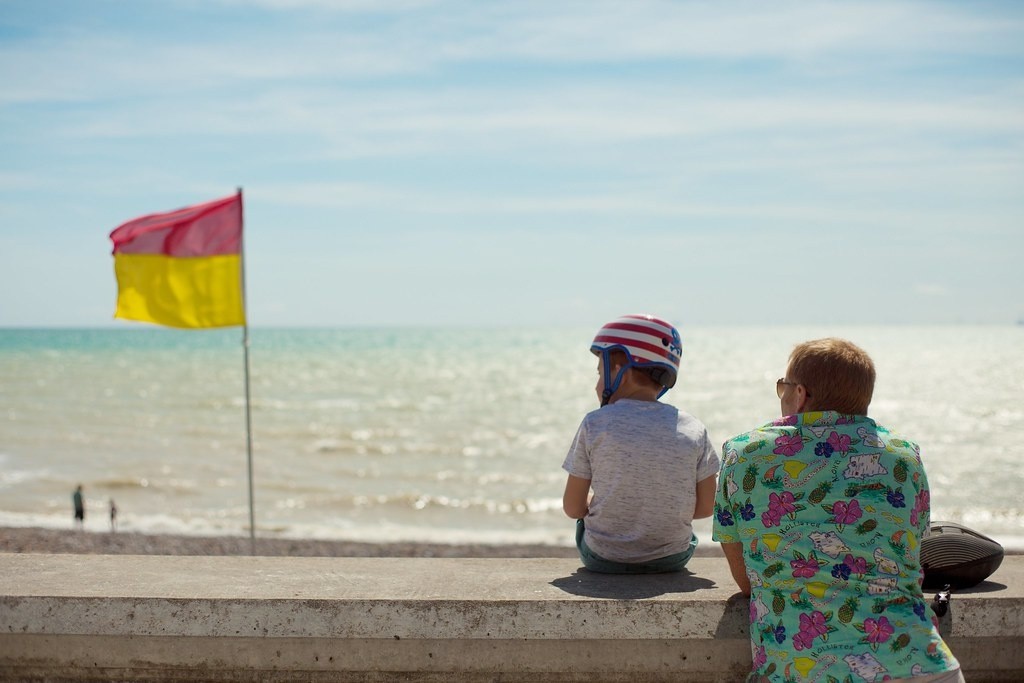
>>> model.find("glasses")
[777,378,811,397]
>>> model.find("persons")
[561,312,720,575]
[73,485,85,531]
[712,336,966,683]
[109,500,117,530]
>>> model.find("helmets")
[590,315,682,388]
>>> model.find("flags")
[108,193,246,328]
